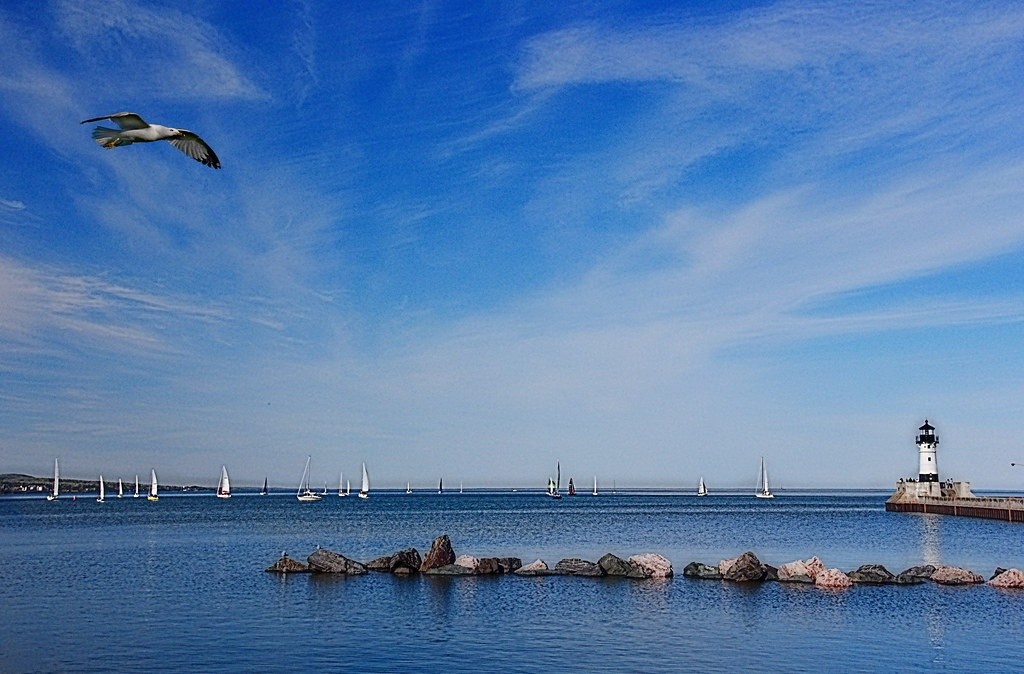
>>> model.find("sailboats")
[567,478,577,496]
[295,456,323,503]
[697,476,708,497]
[216,464,232,499]
[46,457,60,501]
[338,471,346,497]
[357,462,369,499]
[546,461,561,498]
[132,475,139,498]
[592,475,598,496]
[117,477,124,499]
[97,475,105,503]
[406,481,413,494]
[459,481,463,494]
[438,477,443,495]
[754,456,776,499]
[345,480,350,496]
[145,467,159,502]
[259,477,267,496]
[321,481,328,496]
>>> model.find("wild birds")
[80,111,222,170]
